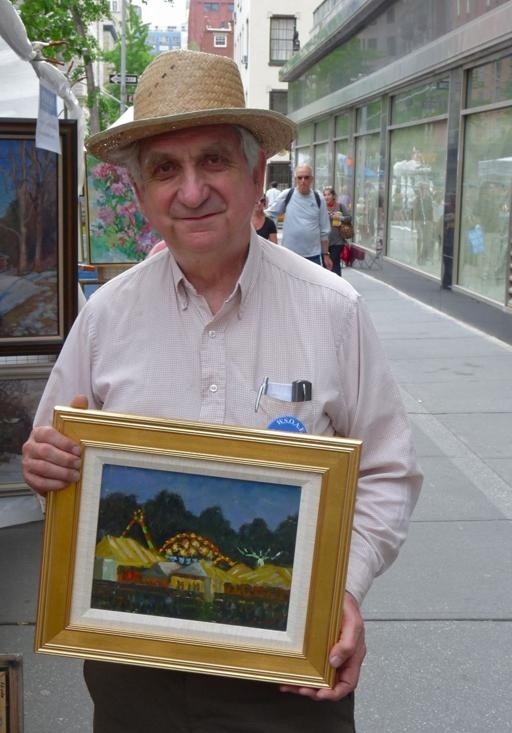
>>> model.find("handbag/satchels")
[338,203,354,239]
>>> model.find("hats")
[84,49,300,170]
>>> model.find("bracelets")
[323,252,330,255]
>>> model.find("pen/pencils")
[79,263,96,271]
[254,376,269,412]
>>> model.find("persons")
[265,181,282,225]
[263,164,333,270]
[323,189,353,277]
[339,182,509,284]
[252,193,277,246]
[21,50,423,733]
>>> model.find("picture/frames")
[0,116,79,357]
[0,360,56,499]
[30,404,361,689]
[84,148,164,267]
